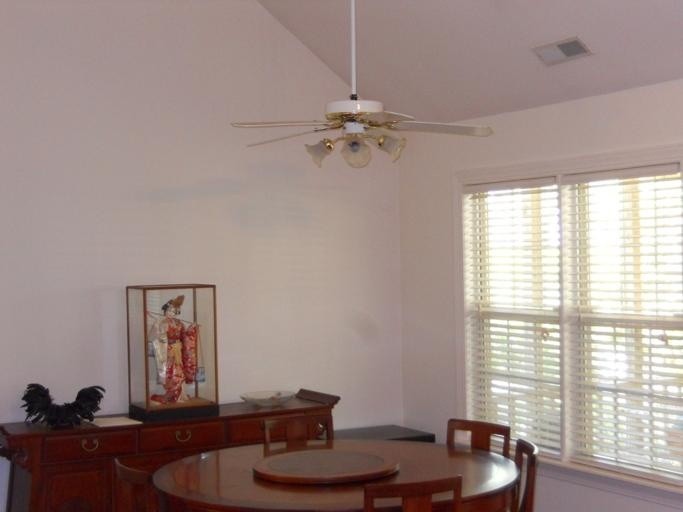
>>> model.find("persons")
[146,300,200,407]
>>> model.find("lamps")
[304,114,407,168]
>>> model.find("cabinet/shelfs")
[0,414,340,510]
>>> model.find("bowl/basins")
[240,390,297,408]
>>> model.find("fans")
[229,1,494,149]
